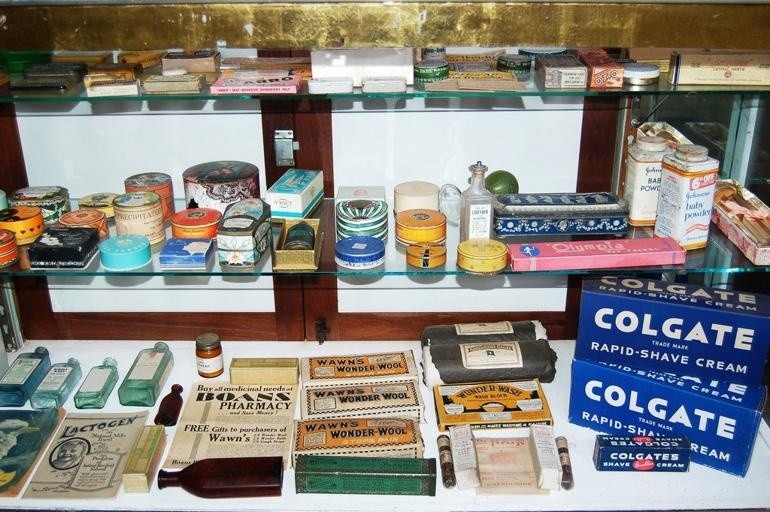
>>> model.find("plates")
[0,48,770,512]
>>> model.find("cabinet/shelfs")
[31,358,82,407]
[654,144,719,250]
[158,456,283,499]
[0,347,51,407]
[118,343,174,407]
[625,138,672,227]
[459,161,494,242]
[196,333,223,377]
[155,384,183,426]
[282,221,315,251]
[73,357,119,409]
